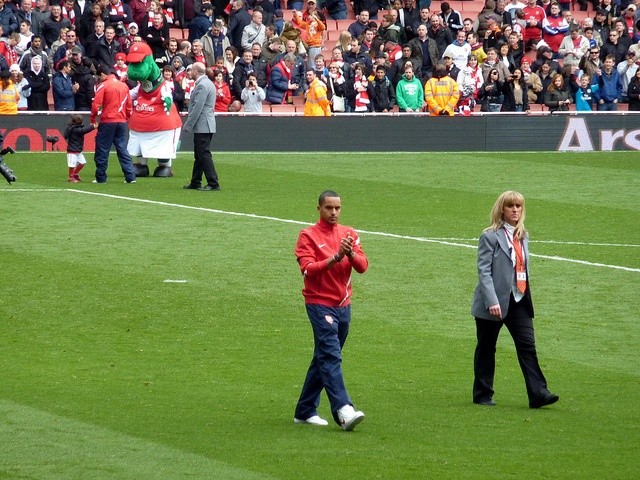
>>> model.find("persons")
[112,53,128,83]
[171,56,186,87]
[117,21,147,52]
[64,114,98,183]
[188,3,216,44]
[90,58,100,84]
[347,10,370,39]
[442,30,471,70]
[503,0,527,19]
[17,0,47,46]
[33,0,51,20]
[382,15,401,43]
[0,70,20,115]
[544,74,574,111]
[154,37,177,72]
[17,20,35,62]
[53,30,76,72]
[86,20,105,66]
[140,0,167,41]
[76,37,86,56]
[467,32,488,67]
[359,28,374,54]
[578,46,604,85]
[413,8,432,34]
[494,0,513,32]
[38,4,73,55]
[483,29,514,90]
[85,5,112,38]
[244,0,259,15]
[628,40,640,62]
[4,0,18,12]
[479,47,511,82]
[428,14,454,60]
[182,64,195,112]
[51,60,80,111]
[315,62,345,112]
[507,31,524,69]
[344,38,373,75]
[60,0,81,31]
[183,62,220,191]
[128,0,152,22]
[201,0,214,24]
[535,63,557,104]
[0,0,19,34]
[267,53,299,104]
[500,69,528,111]
[378,9,407,46]
[370,65,396,112]
[9,64,31,111]
[68,45,97,77]
[250,43,272,93]
[232,50,256,104]
[519,56,543,104]
[569,65,604,111]
[0,24,10,42]
[558,24,590,74]
[470,191,559,408]
[145,13,169,54]
[484,13,503,48]
[272,9,287,36]
[390,43,414,78]
[260,38,286,63]
[496,24,512,51]
[211,55,230,87]
[473,0,503,49]
[398,0,421,33]
[461,18,479,42]
[94,25,123,74]
[384,30,403,64]
[593,9,611,44]
[372,52,396,95]
[527,38,551,60]
[396,66,424,112]
[379,1,395,10]
[542,1,570,59]
[627,66,640,111]
[393,60,419,94]
[259,0,281,27]
[408,25,439,89]
[23,55,50,111]
[346,62,376,112]
[47,27,69,72]
[115,21,127,41]
[579,18,603,48]
[200,21,231,67]
[394,0,403,24]
[226,0,252,57]
[599,29,626,66]
[332,31,352,54]
[316,0,347,20]
[206,15,233,46]
[302,54,328,105]
[161,65,185,111]
[90,65,137,184]
[293,9,327,70]
[124,35,142,66]
[224,46,240,85]
[436,2,464,40]
[212,70,232,112]
[597,0,617,17]
[294,190,368,431]
[590,54,623,111]
[613,0,636,16]
[530,45,566,80]
[241,11,267,52]
[262,24,281,47]
[522,0,547,40]
[456,55,485,116]
[619,4,637,40]
[173,0,203,26]
[169,41,195,68]
[441,52,461,82]
[271,40,307,96]
[73,0,92,34]
[584,27,596,49]
[424,64,459,116]
[253,6,270,27]
[632,18,640,44]
[617,50,639,103]
[367,61,381,85]
[327,48,354,112]
[350,0,379,20]
[72,58,97,111]
[188,38,214,68]
[108,0,133,31]
[302,0,327,30]
[615,18,633,50]
[85,0,109,17]
[0,31,21,67]
[477,68,504,112]
[415,0,431,10]
[536,0,558,17]
[512,8,527,54]
[561,10,572,25]
[18,35,50,74]
[304,68,334,116]
[290,11,306,41]
[287,0,304,10]
[368,21,379,37]
[368,35,388,63]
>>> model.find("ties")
[503,227,526,294]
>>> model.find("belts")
[489,102,502,105]
[515,104,523,106]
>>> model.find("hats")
[9,64,20,72]
[627,50,636,54]
[129,22,138,29]
[610,34,618,36]
[542,47,553,52]
[374,51,386,58]
[627,4,636,11]
[444,53,453,58]
[128,42,155,63]
[202,3,215,10]
[307,0,316,4]
[115,53,126,63]
[72,45,81,54]
[521,57,531,64]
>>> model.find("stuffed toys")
[127,43,183,177]
[279,21,310,69]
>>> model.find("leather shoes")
[202,185,220,191]
[532,394,559,408]
[183,184,201,189]
[477,400,495,405]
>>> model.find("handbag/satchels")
[330,77,345,112]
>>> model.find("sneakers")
[124,180,136,184]
[337,404,365,431]
[74,175,82,181]
[294,416,328,426]
[92,179,105,184]
[68,176,79,183]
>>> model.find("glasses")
[66,35,75,37]
[590,52,598,54]
[33,41,41,42]
[16,40,18,44]
[329,67,336,69]
[382,20,388,23]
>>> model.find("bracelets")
[346,250,353,257]
[334,253,341,263]
